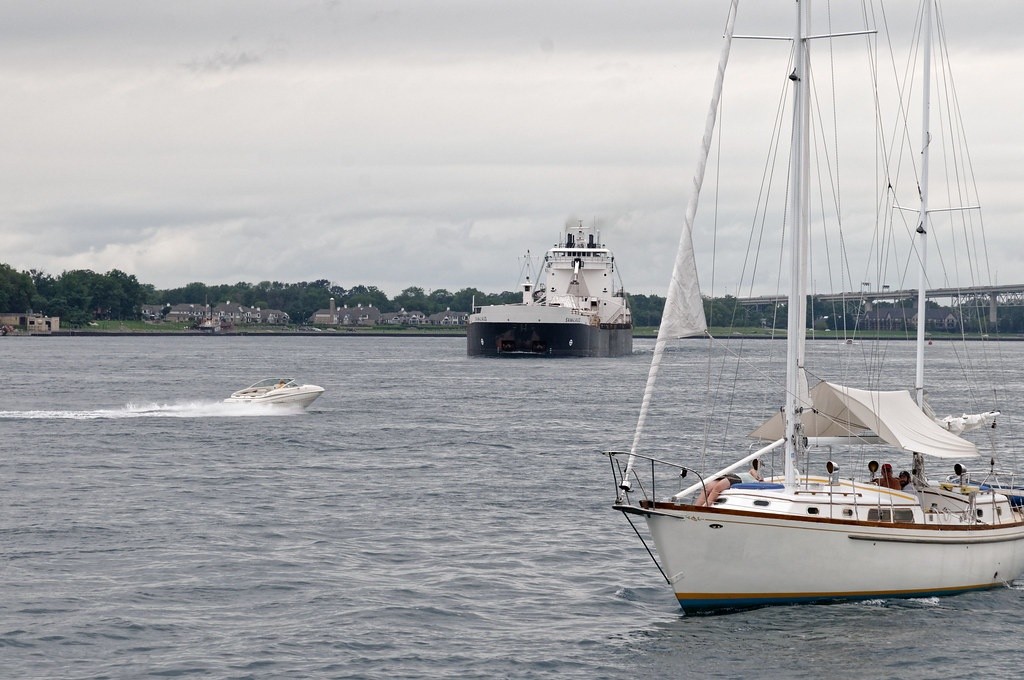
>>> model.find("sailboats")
[594,0,1024,616]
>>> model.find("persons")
[900,471,911,490]
[695,469,763,506]
[870,464,900,490]
[277,378,286,388]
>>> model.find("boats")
[466,218,632,357]
[225,378,326,410]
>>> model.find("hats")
[882,464,892,470]
[899,471,910,478]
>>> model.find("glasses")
[883,469,891,472]
